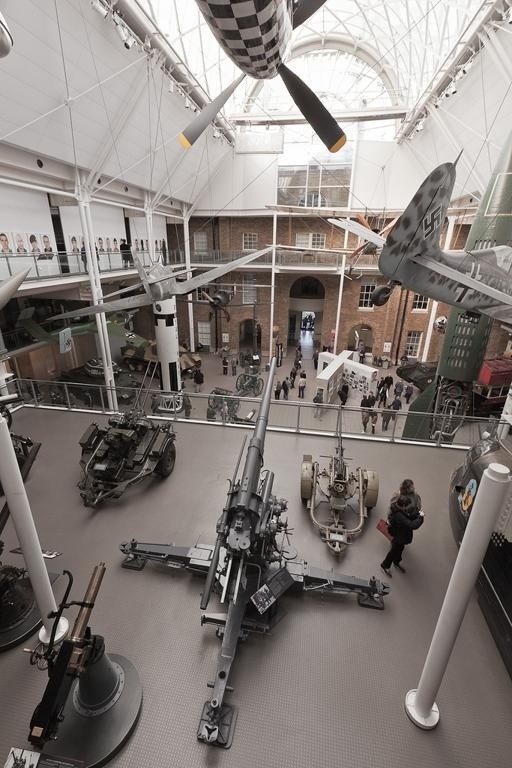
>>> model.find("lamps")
[405,110,428,141]
[167,65,231,139]
[495,0,512,24]
[90,0,143,50]
[434,45,478,109]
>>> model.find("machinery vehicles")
[120,339,201,380]
[59,356,145,405]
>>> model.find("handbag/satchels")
[376,518,395,542]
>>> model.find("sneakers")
[380,564,406,578]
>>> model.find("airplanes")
[15,306,151,355]
[326,163,512,332]
[177,0,347,153]
[45,245,280,323]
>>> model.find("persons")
[221,350,259,378]
[95,242,100,271]
[194,368,204,392]
[0,234,165,259]
[273,345,308,399]
[338,389,346,405]
[391,479,422,512]
[358,344,364,350]
[28,381,40,400]
[312,350,319,369]
[360,348,364,364]
[81,242,88,273]
[379,495,424,579]
[162,241,167,265]
[400,354,408,366]
[313,392,323,420]
[120,239,134,269]
[150,394,160,414]
[183,393,192,418]
[342,382,349,395]
[220,402,228,421]
[206,404,217,422]
[360,375,414,433]
[303,313,316,330]
